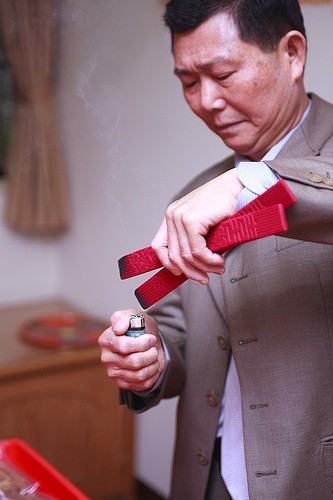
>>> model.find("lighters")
[126,314,146,337]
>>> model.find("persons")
[98,0,333,500]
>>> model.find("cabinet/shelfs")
[0,300,135,500]
